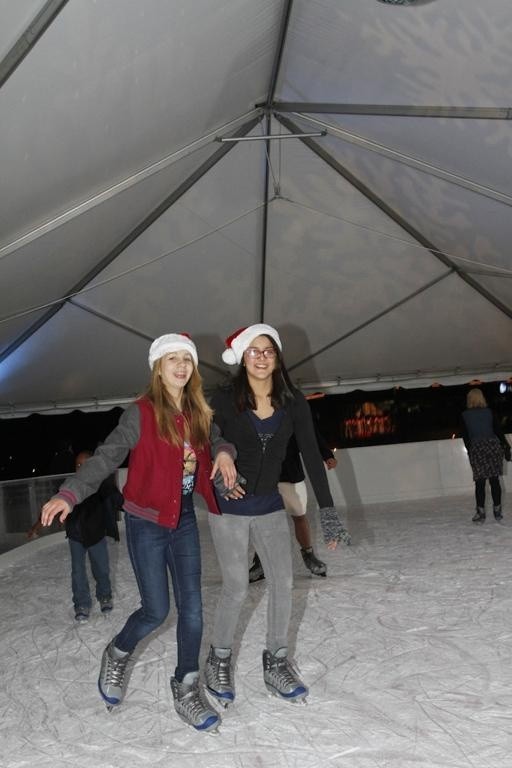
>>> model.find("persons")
[249,413,337,583]
[203,323,351,704]
[40,333,237,732]
[459,388,512,522]
[26,451,125,621]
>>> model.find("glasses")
[245,347,277,360]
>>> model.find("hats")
[221,322,283,366]
[148,332,200,372]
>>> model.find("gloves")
[212,467,247,501]
[316,505,353,549]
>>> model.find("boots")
[471,506,485,521]
[491,503,503,519]
[74,606,90,620]
[203,644,235,703]
[98,597,113,612]
[169,670,222,732]
[97,636,131,704]
[246,552,267,581]
[261,646,308,703]
[300,546,327,575]
